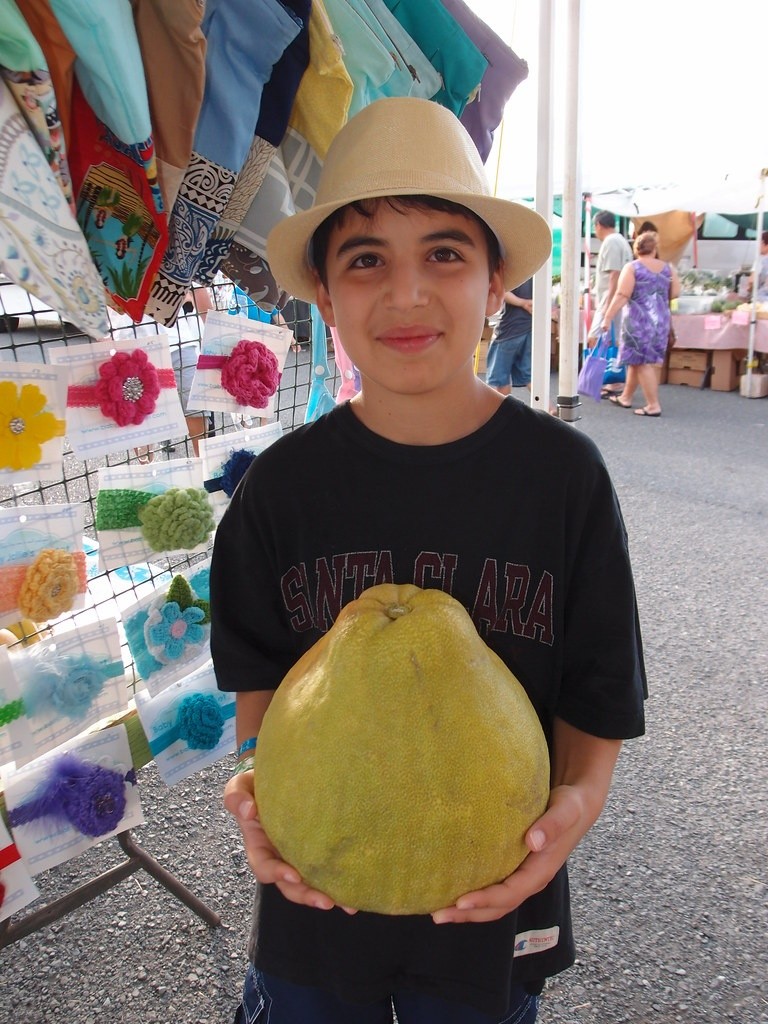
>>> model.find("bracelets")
[234,755,255,774]
[238,737,257,755]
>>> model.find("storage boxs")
[472,319,559,374]
[678,294,714,314]
[638,349,747,392]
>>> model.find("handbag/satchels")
[583,320,627,385]
[577,329,610,403]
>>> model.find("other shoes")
[158,439,176,452]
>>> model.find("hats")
[264,95,551,304]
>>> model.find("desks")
[579,307,768,354]
[0,561,223,951]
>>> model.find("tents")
[586,175,768,398]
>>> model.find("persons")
[587,210,634,398]
[486,277,557,417]
[633,221,658,259]
[601,233,679,417]
[98,282,214,466]
[748,232,768,302]
[228,276,301,426]
[211,97,649,1024]
[215,285,227,308]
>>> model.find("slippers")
[608,394,631,408]
[600,386,623,399]
[633,407,662,417]
